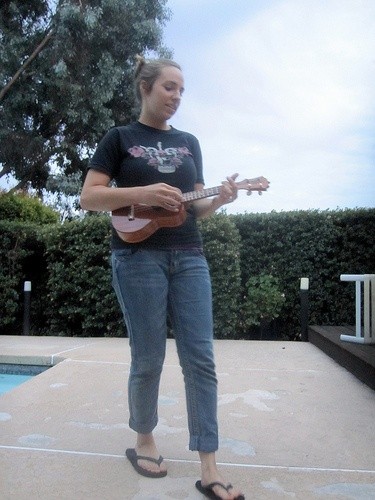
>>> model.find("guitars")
[110,175,271,244]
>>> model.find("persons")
[80,60,247,500]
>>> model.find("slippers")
[194,479,245,500]
[124,446,169,480]
